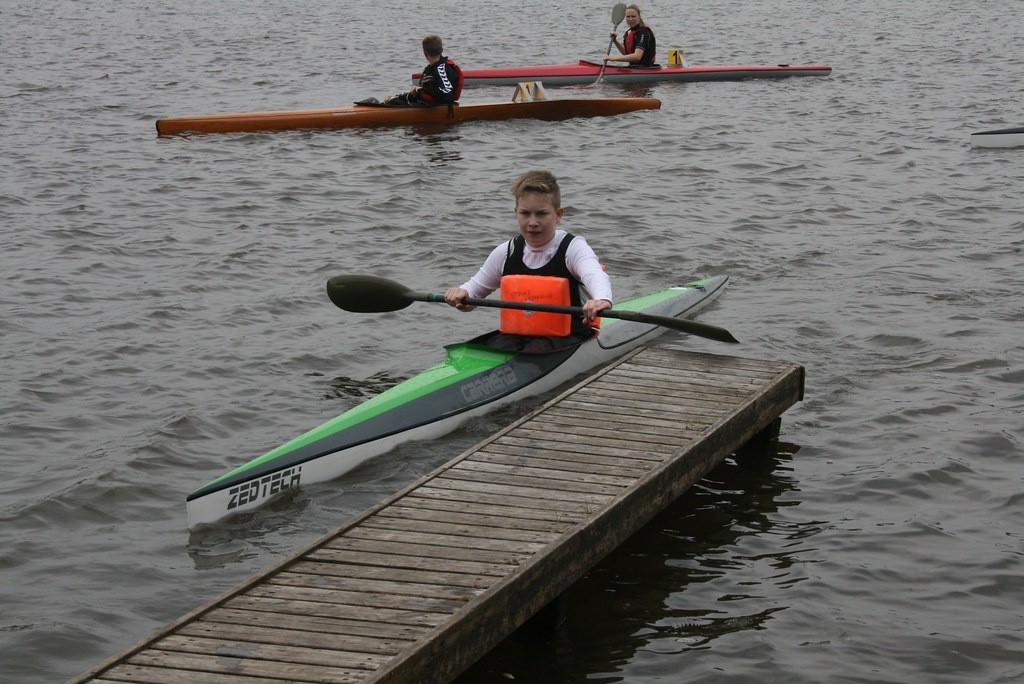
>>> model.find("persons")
[603,5,656,66]
[444,170,613,352]
[384,35,464,106]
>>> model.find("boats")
[184,273,732,531]
[153,82,661,135]
[409,50,833,87]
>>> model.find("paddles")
[325,273,742,346]
[595,3,627,85]
